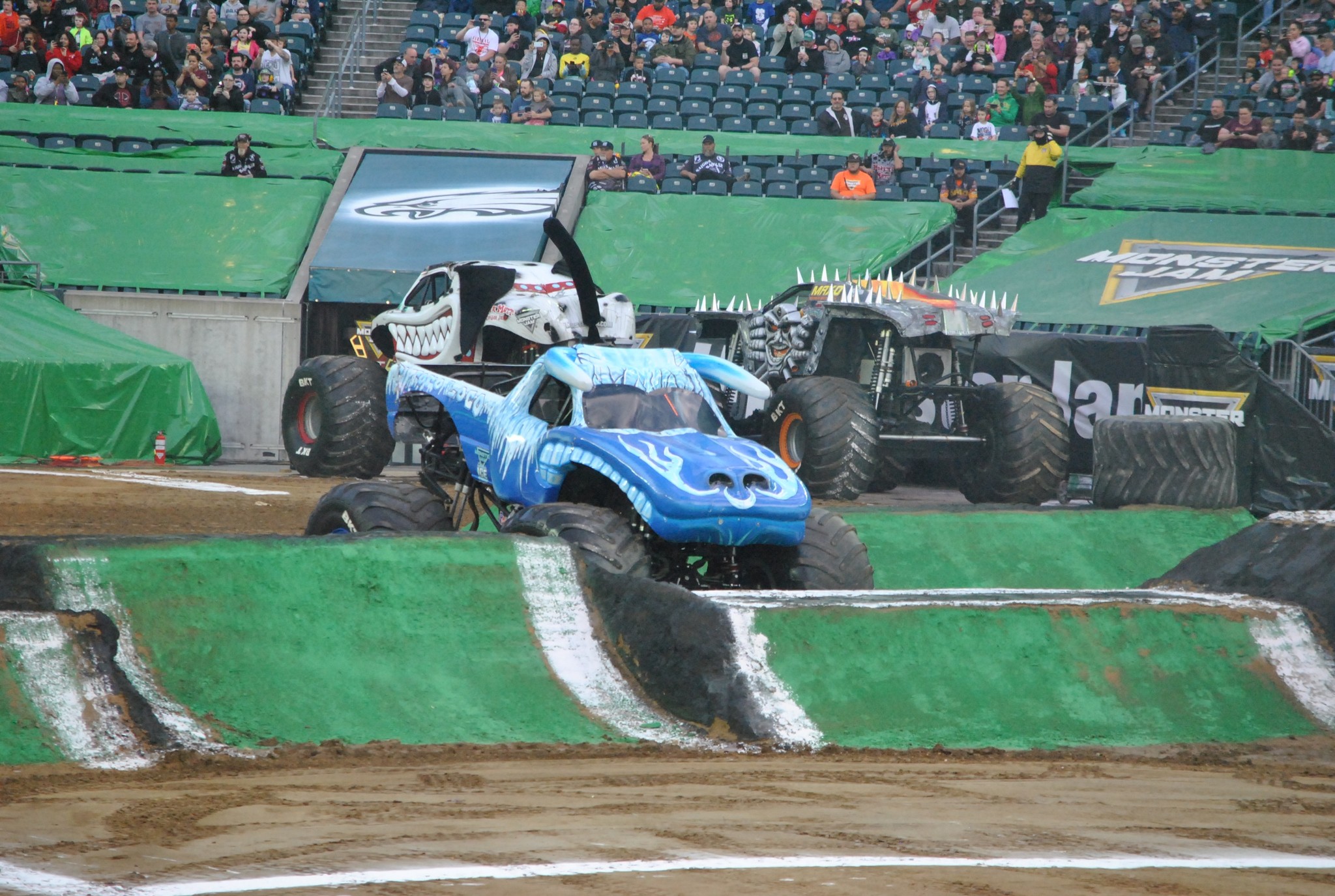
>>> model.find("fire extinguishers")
[150,430,167,464]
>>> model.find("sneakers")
[737,172,750,182]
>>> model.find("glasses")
[832,97,842,100]
[478,18,490,22]
[223,78,233,83]
[569,22,579,26]
[18,17,30,21]
[1311,77,1323,82]
[392,63,403,66]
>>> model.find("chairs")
[15,135,267,154]
[375,0,1335,274]
[0,0,339,115]
[1011,320,1267,364]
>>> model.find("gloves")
[1046,130,1053,140]
[1010,177,1020,194]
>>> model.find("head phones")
[913,36,929,47]
[949,160,970,173]
[879,138,896,151]
[658,30,672,42]
[973,39,989,52]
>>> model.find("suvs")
[731,280,1074,508]
[282,257,877,602]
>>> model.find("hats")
[1032,125,1048,134]
[731,22,744,31]
[883,138,894,146]
[590,140,614,150]
[260,68,271,75]
[952,159,966,169]
[671,19,687,28]
[236,134,249,142]
[935,0,1185,48]
[266,32,280,42]
[114,65,130,77]
[1309,70,1323,78]
[620,21,633,29]
[506,17,518,24]
[906,23,920,32]
[702,135,714,143]
[848,154,861,162]
[395,39,449,80]
[552,0,565,7]
[110,0,121,8]
[804,30,815,41]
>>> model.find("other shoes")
[456,100,465,107]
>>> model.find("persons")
[681,135,751,184]
[1004,122,1064,229]
[0,0,336,115]
[221,131,269,178]
[872,139,902,185]
[375,0,1335,153]
[829,152,876,201]
[938,159,978,245]
[584,140,628,192]
[627,134,667,189]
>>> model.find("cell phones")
[533,42,543,47]
[570,61,574,66]
[24,40,31,52]
[602,42,613,47]
[184,60,190,68]
[203,21,209,30]
[429,49,440,55]
[615,7,620,13]
[990,104,997,109]
[1234,132,1239,136]
[218,81,223,87]
[525,107,531,112]
[63,71,67,77]
[491,68,497,73]
[514,29,519,36]
[56,42,63,47]
[383,69,387,72]
[800,46,805,54]
[473,21,483,26]
